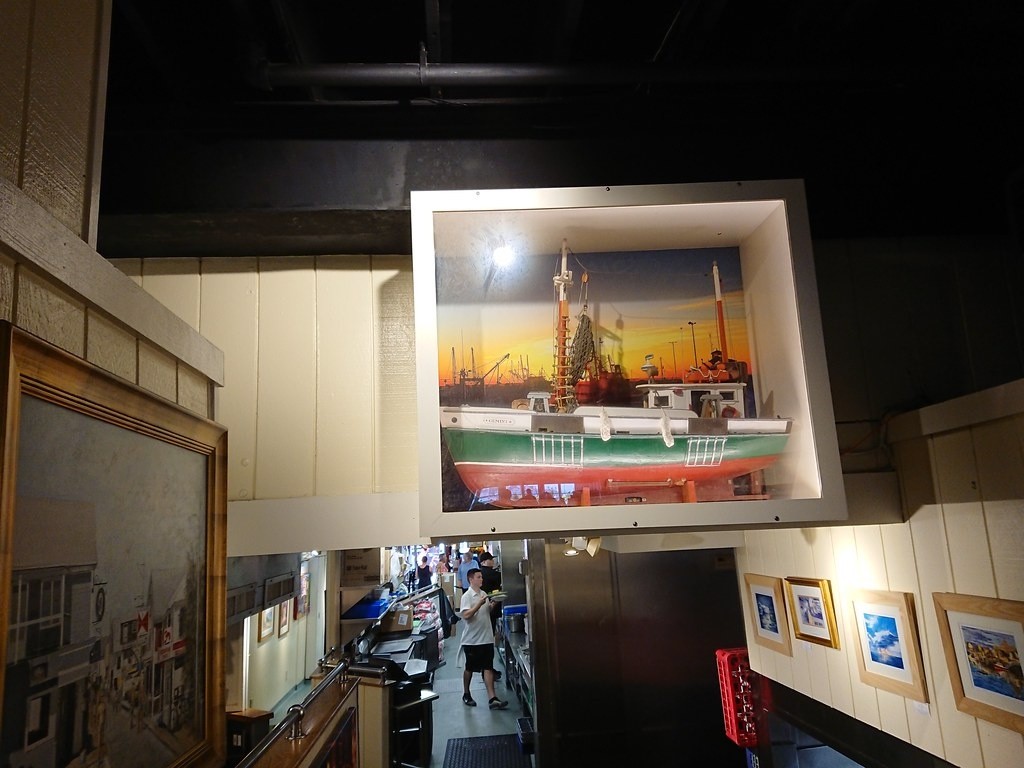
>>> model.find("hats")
[479,552,498,563]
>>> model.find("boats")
[439,236,794,509]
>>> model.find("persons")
[415,551,503,682]
[459,568,508,709]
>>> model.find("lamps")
[563,536,601,557]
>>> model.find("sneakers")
[462,695,477,706]
[489,697,509,710]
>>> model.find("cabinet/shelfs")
[364,623,439,768]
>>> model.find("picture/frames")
[931,592,1023,735]
[0,318,229,768]
[258,607,275,642]
[783,577,839,649]
[743,572,793,657]
[278,598,290,639]
[308,707,360,768]
[293,574,312,619]
[848,589,929,703]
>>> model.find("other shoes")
[482,669,502,680]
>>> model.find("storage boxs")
[381,605,414,633]
[516,717,536,756]
[716,648,759,768]
[441,571,463,637]
